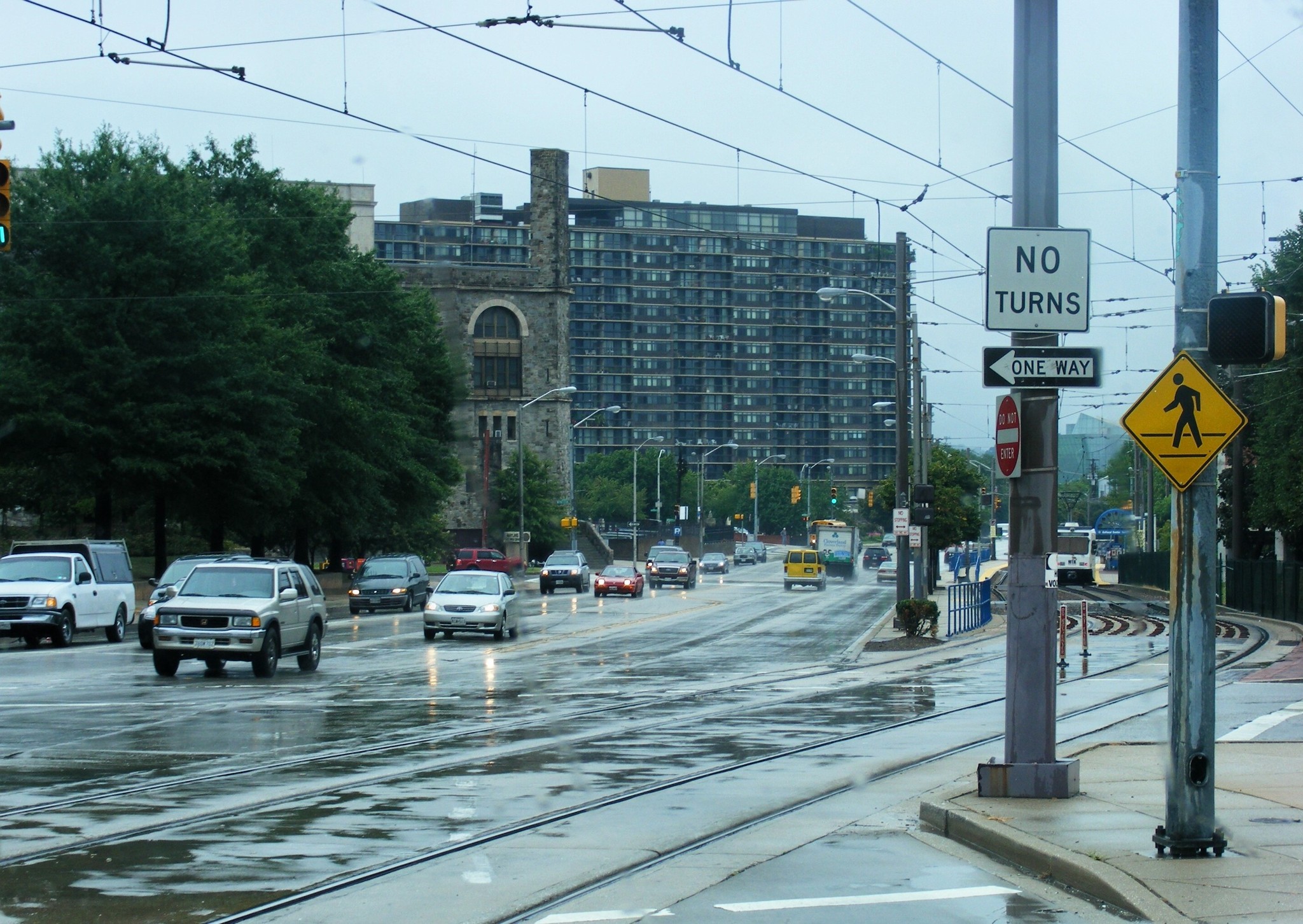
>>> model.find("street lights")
[753,454,786,542]
[816,287,927,601]
[807,459,835,547]
[568,405,739,569]
[800,464,808,486]
[826,466,834,519]
[517,386,577,577]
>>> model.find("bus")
[1057,523,1097,586]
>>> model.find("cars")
[423,570,518,640]
[594,542,767,598]
[782,518,991,592]
[138,553,255,649]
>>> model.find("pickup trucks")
[0,538,135,647]
[152,556,328,678]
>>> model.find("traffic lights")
[795,486,801,501]
[674,505,680,515]
[1208,291,1285,366]
[830,488,837,504]
[802,517,806,521]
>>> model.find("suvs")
[446,548,526,576]
[539,550,591,594]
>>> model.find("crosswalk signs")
[1119,350,1248,493]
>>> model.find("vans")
[348,553,430,614]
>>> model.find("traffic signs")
[981,346,1106,388]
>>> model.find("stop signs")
[994,393,1021,479]
